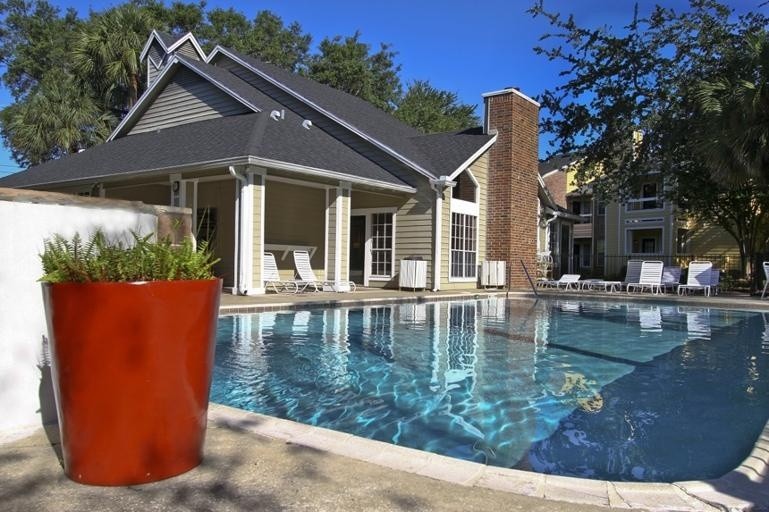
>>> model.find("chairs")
[760,262,769,299]
[540,257,725,298]
[261,248,357,294]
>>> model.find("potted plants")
[37,231,224,484]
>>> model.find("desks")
[480,260,507,289]
[399,259,427,292]
